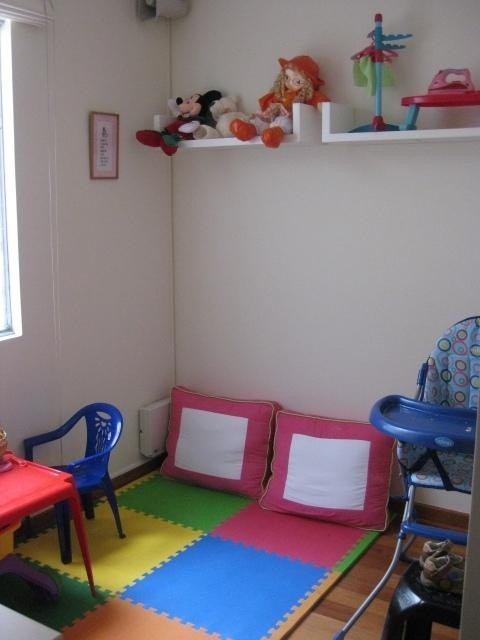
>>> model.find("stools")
[380,561,465,640]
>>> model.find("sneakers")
[421,539,464,595]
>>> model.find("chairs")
[23,402,126,564]
[333,315,480,640]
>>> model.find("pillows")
[258,410,395,531]
[161,386,279,500]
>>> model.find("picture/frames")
[89,111,118,180]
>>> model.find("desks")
[0,454,96,599]
